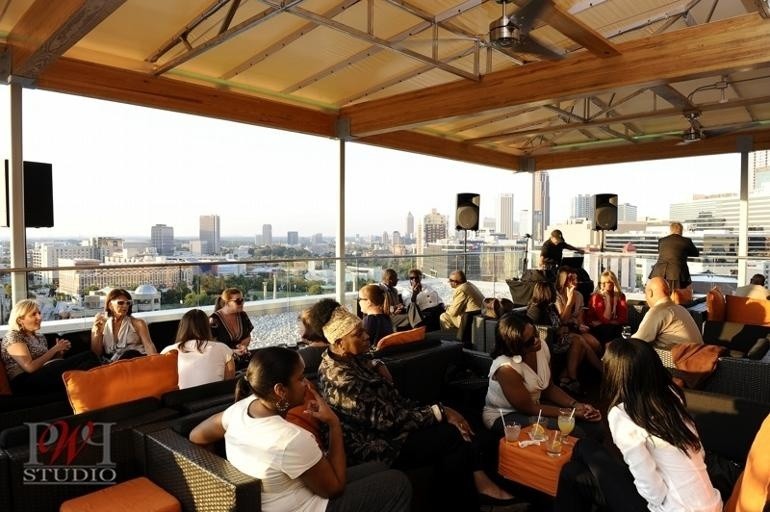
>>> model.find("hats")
[323,306,362,344]
[551,230,564,242]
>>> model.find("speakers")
[456,193,480,229]
[595,194,618,230]
[5,159,53,227]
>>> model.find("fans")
[620,98,760,146]
[395,0,569,67]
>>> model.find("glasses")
[357,297,367,302]
[111,300,132,305]
[231,298,243,305]
[522,325,539,347]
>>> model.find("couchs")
[0,280,770,512]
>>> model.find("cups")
[558,407,575,443]
[98,312,109,324]
[528,415,548,440]
[621,325,632,340]
[504,421,522,444]
[544,431,563,457]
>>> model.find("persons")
[648,222,699,295]
[188,347,412,512]
[296,264,724,512]
[91,289,158,364]
[159,310,235,390]
[734,274,770,301]
[1,298,101,396]
[208,287,254,376]
[539,230,585,270]
